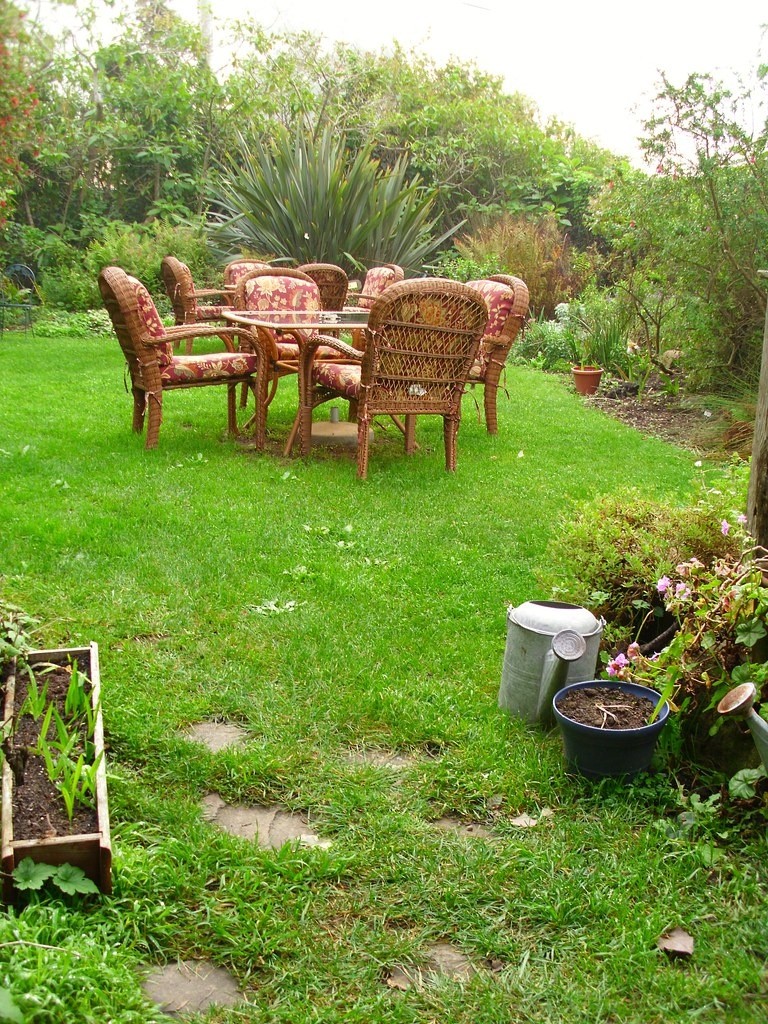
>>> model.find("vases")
[553,679,670,779]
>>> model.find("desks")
[224,279,363,306]
[223,309,420,456]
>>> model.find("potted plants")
[571,357,604,395]
[2,640,114,908]
[531,484,753,656]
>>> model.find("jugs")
[717,681,768,773]
[499,601,607,727]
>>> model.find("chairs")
[98,266,268,453]
[464,274,530,435]
[220,257,407,409]
[297,277,489,479]
[0,264,36,339]
[160,256,236,354]
